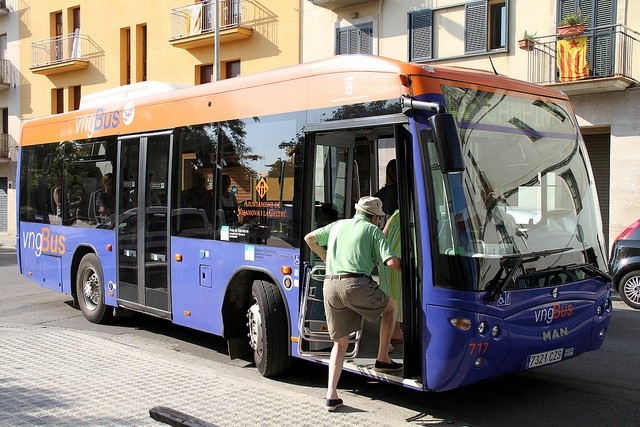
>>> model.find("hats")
[355,196,385,216]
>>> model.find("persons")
[380,209,404,352]
[52,175,82,225]
[217,174,239,223]
[304,196,404,411]
[94,172,115,229]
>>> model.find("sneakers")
[374,360,403,371]
[325,398,343,412]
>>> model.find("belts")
[325,273,372,280]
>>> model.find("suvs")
[608,218,640,308]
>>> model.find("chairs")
[171,207,214,238]
[50,185,60,214]
[87,191,96,226]
[294,266,365,360]
[93,188,105,226]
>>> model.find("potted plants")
[518,30,539,51]
[556,14,589,37]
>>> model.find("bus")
[14,53,613,391]
